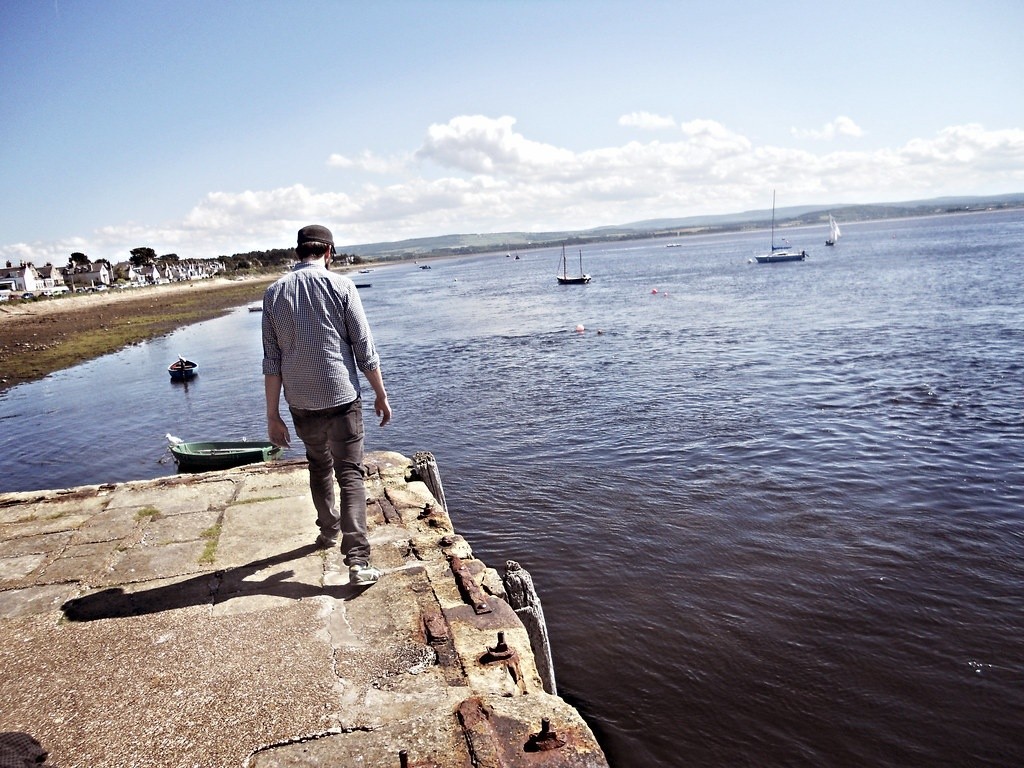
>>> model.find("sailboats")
[826,214,841,246]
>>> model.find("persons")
[260,224,395,594]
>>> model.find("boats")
[168,442,284,466]
[556,242,591,284]
[248,307,262,311]
[755,189,809,263]
[168,354,198,378]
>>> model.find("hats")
[297,225,336,254]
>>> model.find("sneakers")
[320,532,338,546]
[349,561,384,585]
